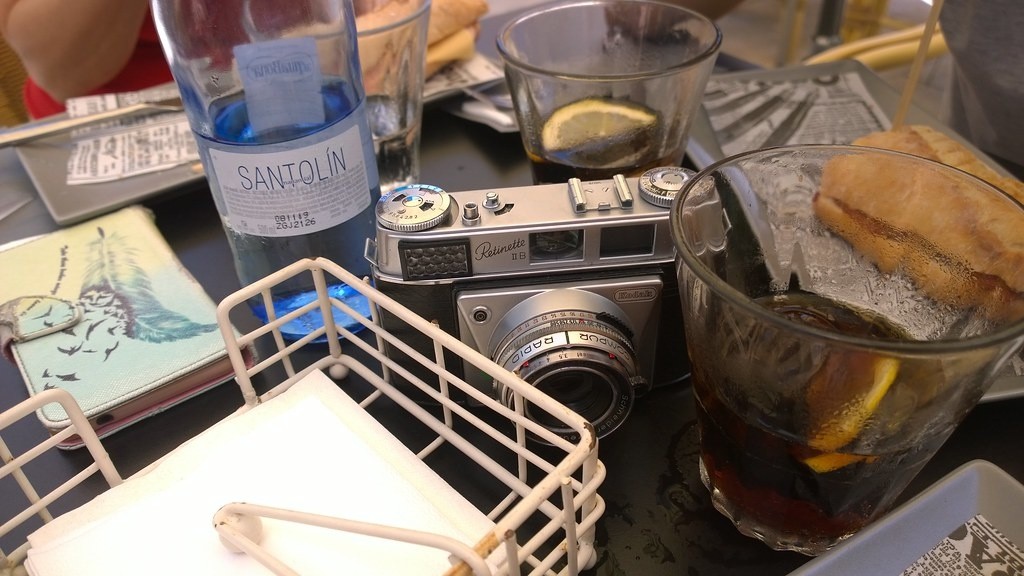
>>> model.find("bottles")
[151,0,382,344]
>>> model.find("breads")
[815,123,1024,324]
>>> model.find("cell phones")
[1,203,260,451]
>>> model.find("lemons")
[807,350,900,473]
[544,100,657,167]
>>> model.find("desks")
[0,1,1024,576]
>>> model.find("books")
[0,203,259,450]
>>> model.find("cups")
[354,0,432,197]
[670,143,1024,558]
[496,0,723,184]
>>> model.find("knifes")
[0,96,187,147]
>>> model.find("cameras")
[364,168,728,446]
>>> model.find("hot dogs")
[232,1,488,97]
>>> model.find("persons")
[0,0,373,122]
[603,0,1024,182]
